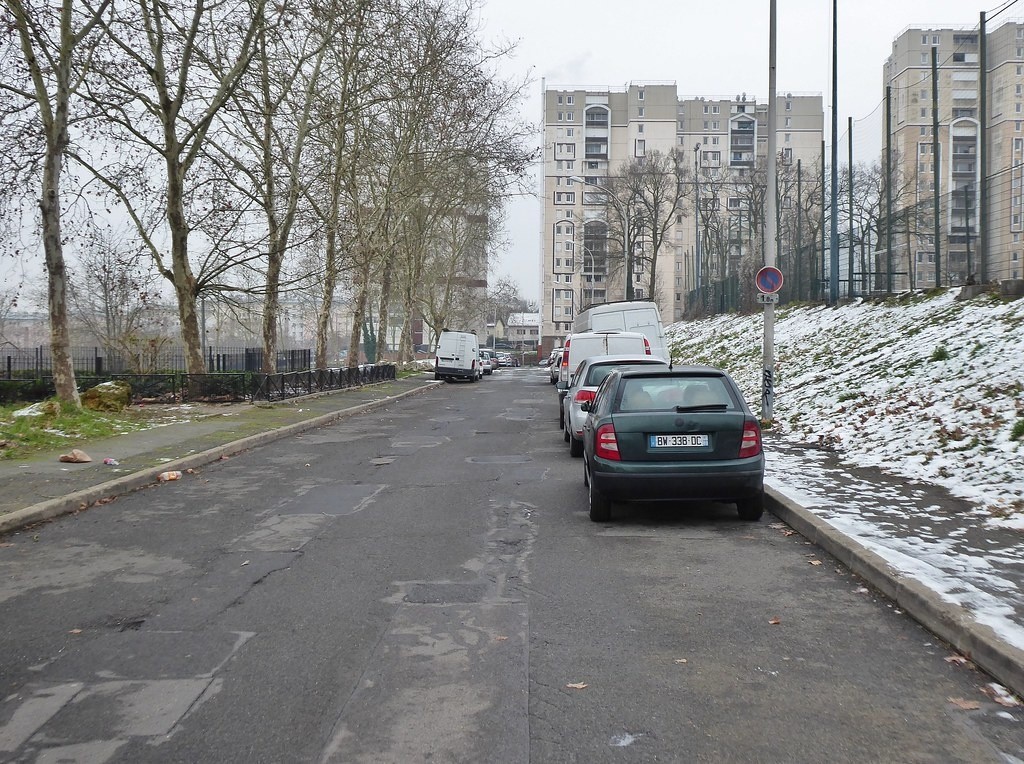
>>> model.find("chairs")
[693,391,720,406]
[684,384,708,402]
[592,369,606,385]
[629,393,654,409]
[624,384,643,403]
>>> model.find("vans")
[434,328,481,384]
[557,331,651,430]
[573,298,671,365]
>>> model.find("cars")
[479,348,520,379]
[556,354,668,459]
[580,366,765,524]
[546,348,564,385]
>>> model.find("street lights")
[565,239,595,303]
[554,281,580,311]
